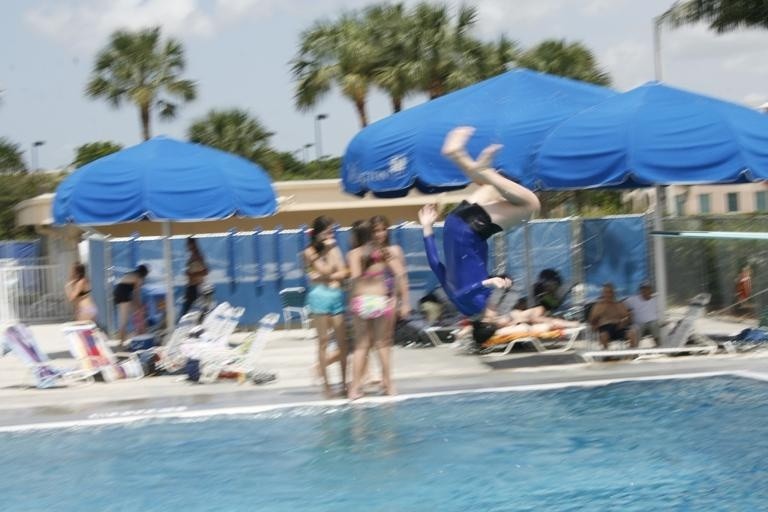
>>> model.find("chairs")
[394,289,718,368]
[5,301,281,386]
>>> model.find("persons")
[64,263,100,323]
[418,125,541,346]
[589,280,664,353]
[422,272,579,342]
[175,235,210,324]
[112,264,149,339]
[303,216,412,401]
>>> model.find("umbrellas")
[520,81,768,326]
[334,69,618,312]
[52,134,280,331]
